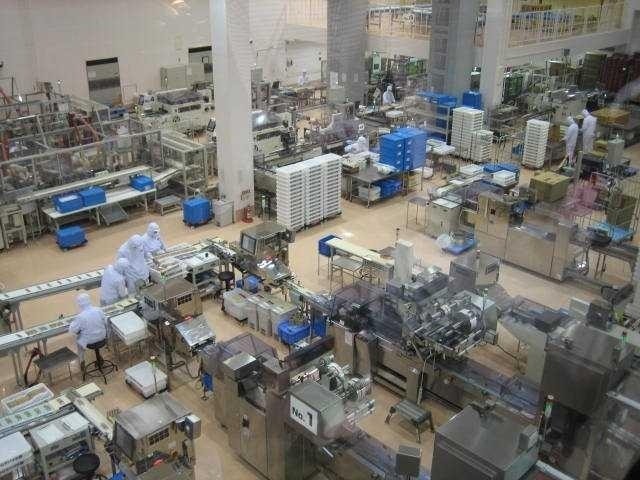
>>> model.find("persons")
[9,142,28,152]
[117,234,151,295]
[560,116,579,165]
[69,293,112,372]
[142,222,169,262]
[298,71,312,86]
[382,85,396,106]
[351,137,367,153]
[100,258,130,344]
[578,109,597,155]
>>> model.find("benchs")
[384,397,435,443]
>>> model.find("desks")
[33,346,78,385]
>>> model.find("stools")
[214,271,235,311]
[73,453,109,480]
[83,339,118,384]
